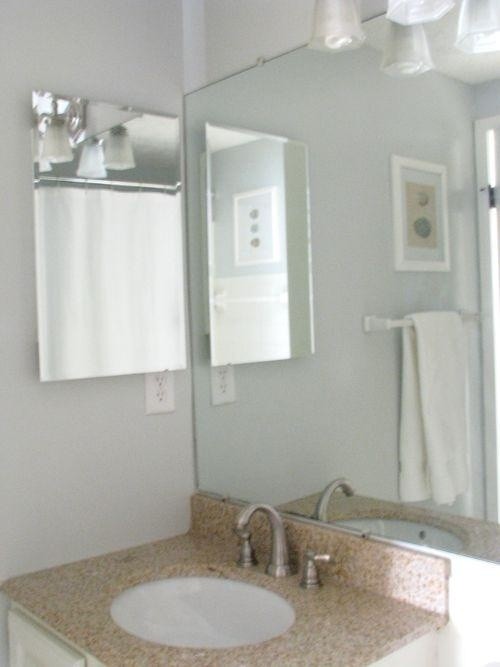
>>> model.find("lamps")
[380,0,500,76]
[307,0,454,55]
[31,91,136,178]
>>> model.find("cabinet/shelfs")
[8,598,102,666]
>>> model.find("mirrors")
[32,90,188,383]
[203,121,314,367]
[185,0,500,565]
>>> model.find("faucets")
[235,503,291,578]
[314,478,354,523]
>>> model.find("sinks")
[110,571,295,649]
[333,510,469,554]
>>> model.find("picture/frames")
[390,154,451,273]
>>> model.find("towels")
[398,309,471,508]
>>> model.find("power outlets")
[210,366,236,407]
[144,371,176,416]
